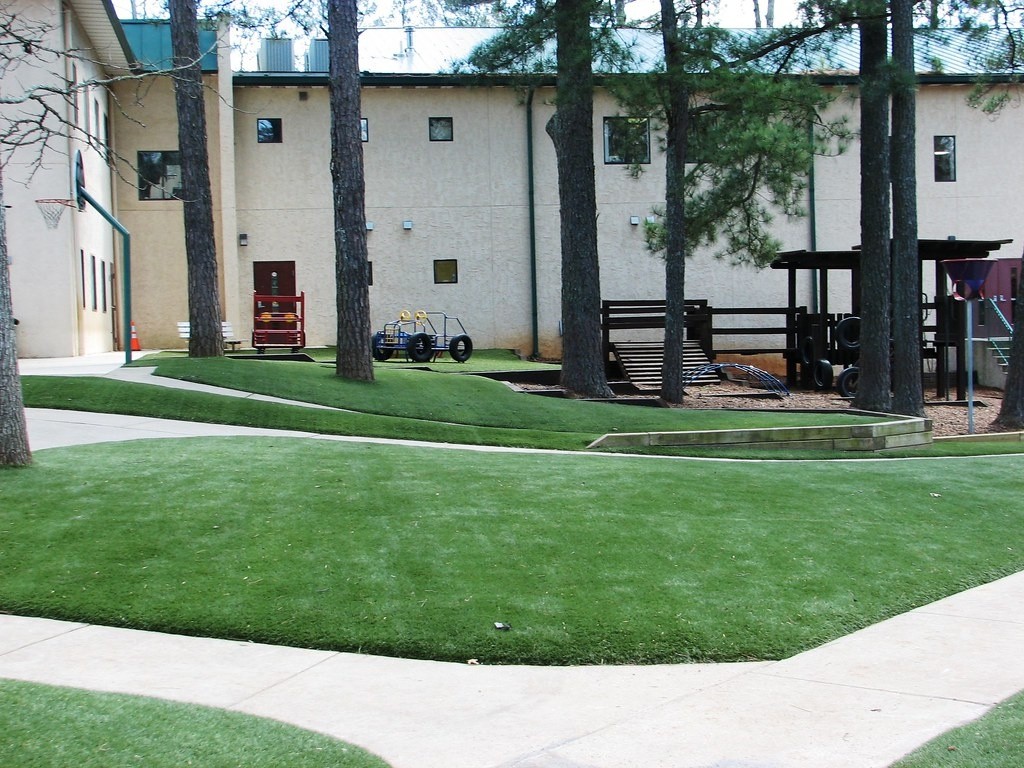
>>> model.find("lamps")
[240,234,248,246]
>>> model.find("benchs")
[177,322,241,353]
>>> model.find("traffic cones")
[130,322,141,352]
